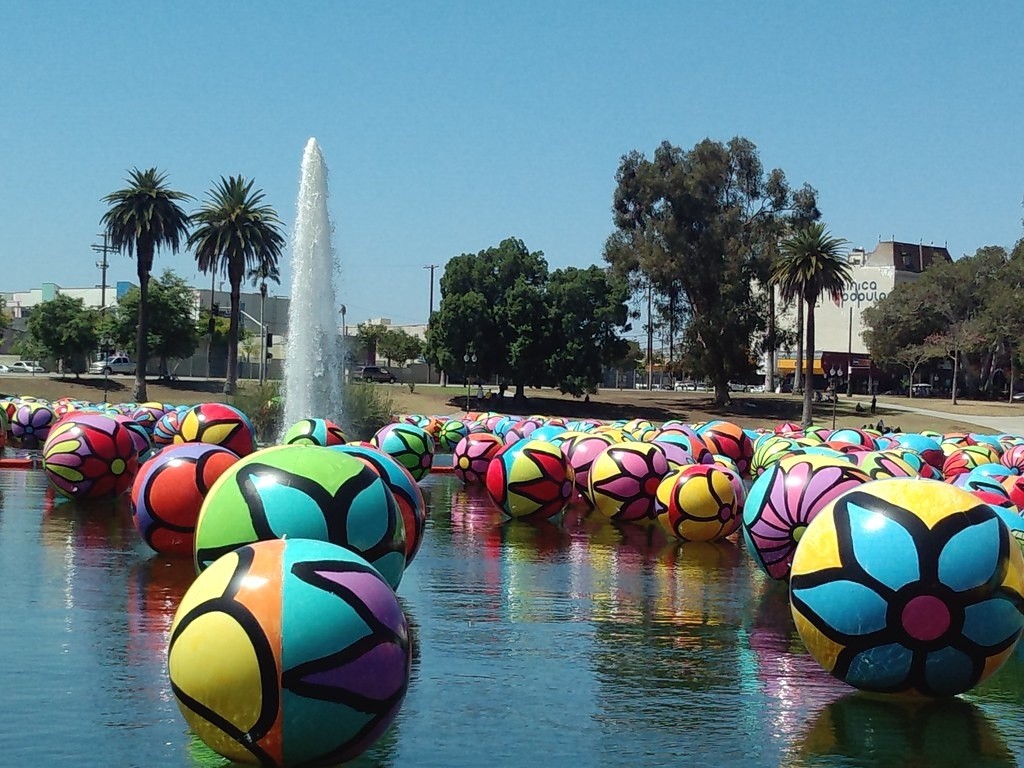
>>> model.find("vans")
[354,365,398,384]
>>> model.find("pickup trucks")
[87,352,147,374]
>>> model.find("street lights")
[465,340,477,411]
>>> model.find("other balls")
[0,388,1023,768]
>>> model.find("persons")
[856,402,864,411]
[483,389,492,410]
[861,419,902,433]
[477,387,482,406]
[814,389,838,403]
[869,395,877,412]
[906,385,1010,401]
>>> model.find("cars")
[633,377,766,393]
[774,384,792,393]
[1012,392,1023,401]
[0,365,10,372]
[911,384,933,397]
[9,361,45,373]
